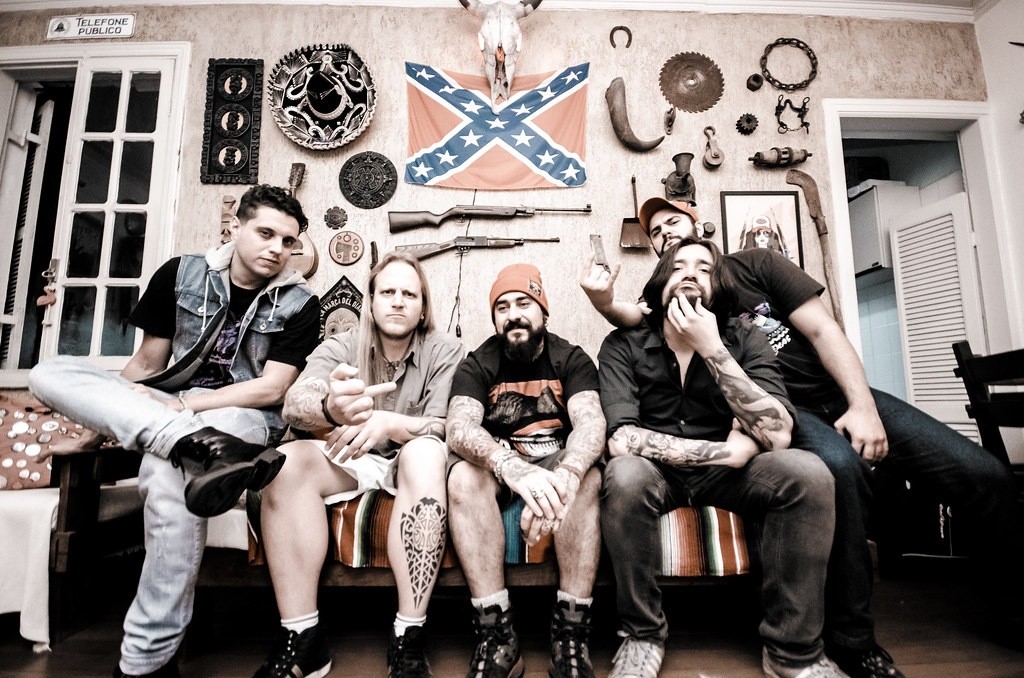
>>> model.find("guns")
[388,203,592,233]
[395,236,560,260]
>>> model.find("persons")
[443,263,599,678]
[29,184,321,678]
[599,238,854,678]
[580,197,1024,678]
[251,252,464,678]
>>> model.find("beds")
[0,395,146,656]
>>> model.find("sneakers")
[387,622,433,678]
[824,645,906,678]
[608,630,665,678]
[763,646,851,678]
[549,599,594,678]
[253,623,336,678]
[466,604,525,678]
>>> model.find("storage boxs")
[847,179,907,199]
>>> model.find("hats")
[490,264,549,316]
[639,197,698,237]
[267,44,379,151]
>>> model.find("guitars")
[285,162,319,279]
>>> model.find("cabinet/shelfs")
[847,184,922,278]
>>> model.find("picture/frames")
[719,189,805,272]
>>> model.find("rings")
[532,488,544,498]
[603,263,612,275]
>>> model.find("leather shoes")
[169,427,286,518]
[112,657,180,678]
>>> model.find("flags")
[404,61,589,189]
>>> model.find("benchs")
[171,481,877,666]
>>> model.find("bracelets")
[322,394,343,428]
[176,390,187,412]
[552,464,584,482]
[493,450,520,483]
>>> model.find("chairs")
[950,339,1024,496]
